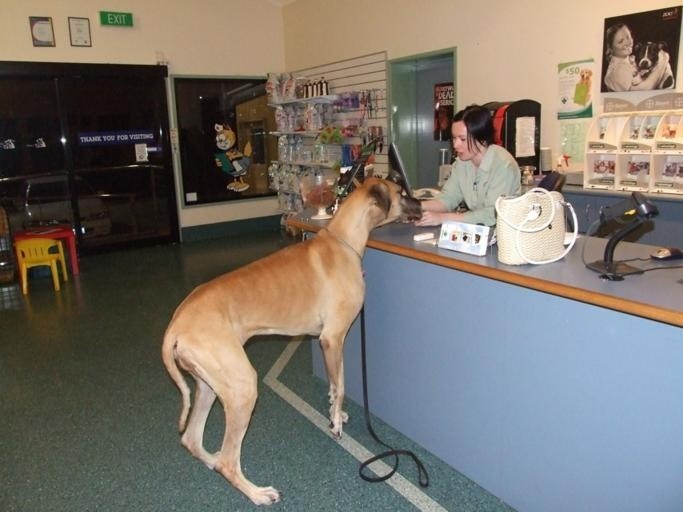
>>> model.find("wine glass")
[298,175,338,220]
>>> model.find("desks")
[13,225,79,284]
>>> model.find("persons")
[603,22,670,92]
[414,104,522,226]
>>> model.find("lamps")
[578,190,660,283]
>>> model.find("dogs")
[631,42,674,89]
[161,176,422,506]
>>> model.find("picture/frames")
[29,16,56,47]
[67,16,93,47]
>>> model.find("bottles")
[540,147,552,175]
[438,148,449,167]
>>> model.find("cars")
[21,169,113,249]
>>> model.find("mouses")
[650,247,683,261]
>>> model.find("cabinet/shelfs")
[562,183,683,248]
[268,94,374,219]
[586,110,683,195]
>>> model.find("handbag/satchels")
[495,188,578,265]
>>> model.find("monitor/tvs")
[388,143,413,198]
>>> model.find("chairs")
[12,238,68,294]
